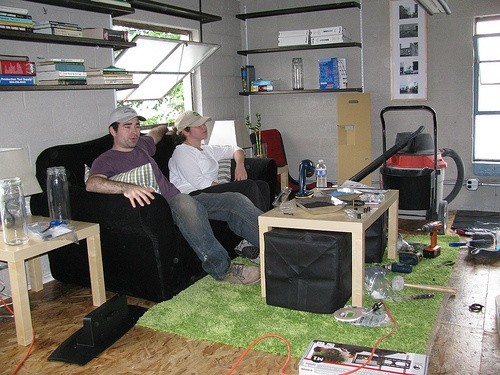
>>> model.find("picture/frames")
[388,0,428,102]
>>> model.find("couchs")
[30,133,279,303]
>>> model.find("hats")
[108,107,146,129]
[175,111,212,135]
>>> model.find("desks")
[258,187,400,314]
[0,215,106,345]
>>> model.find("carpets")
[138,235,461,362]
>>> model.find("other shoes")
[235,239,260,259]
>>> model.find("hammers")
[392,275,458,293]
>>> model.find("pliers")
[437,260,455,268]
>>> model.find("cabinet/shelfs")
[235,0,363,93]
[0,0,140,90]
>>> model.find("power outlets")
[466,179,478,192]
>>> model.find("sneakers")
[224,263,261,285]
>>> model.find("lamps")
[414,0,452,16]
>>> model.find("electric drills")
[415,221,443,258]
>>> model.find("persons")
[86,107,266,285]
[168,110,271,259]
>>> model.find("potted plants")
[246,112,268,159]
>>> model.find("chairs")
[250,129,335,201]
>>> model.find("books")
[88,64,133,85]
[0,54,35,86]
[0,5,32,32]
[278,30,309,47]
[83,27,129,42]
[32,18,83,37]
[310,26,352,46]
[36,56,88,85]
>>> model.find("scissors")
[41,220,65,234]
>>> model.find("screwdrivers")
[342,199,380,206]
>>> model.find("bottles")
[315,159,327,188]
[291,58,304,91]
[47,166,71,228]
[0,176,29,246]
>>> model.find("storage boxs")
[318,58,348,90]
[277,26,351,46]
